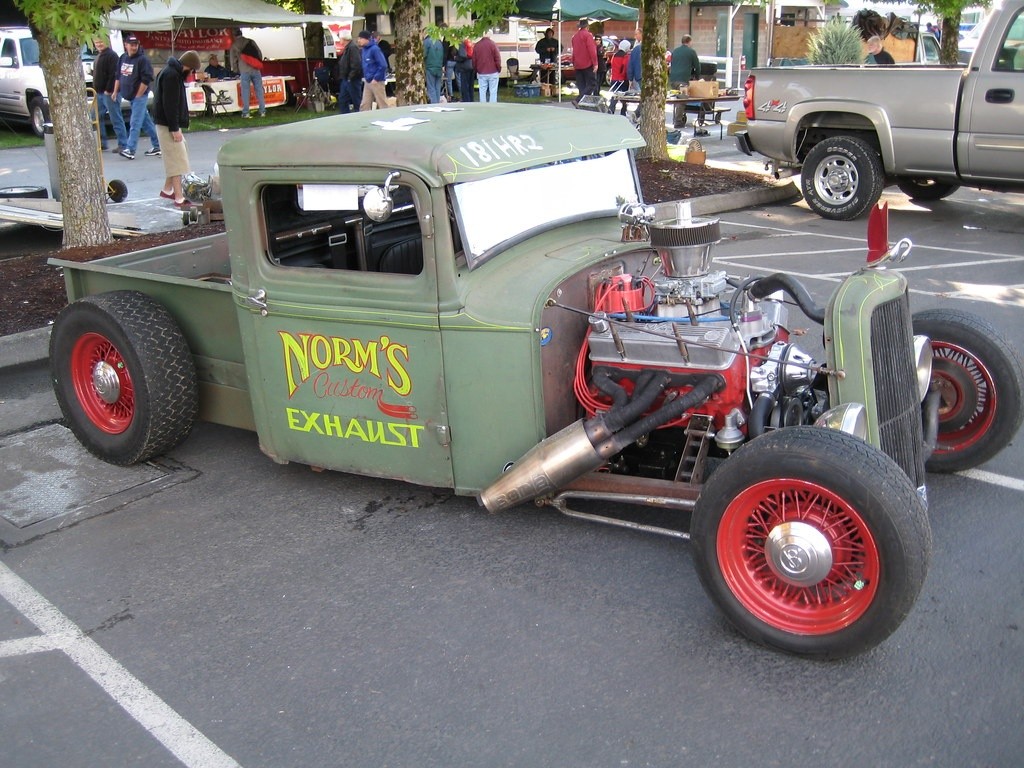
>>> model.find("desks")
[613,95,740,142]
[186,76,296,114]
[362,77,396,83]
[530,65,576,84]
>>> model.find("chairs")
[201,84,234,124]
[376,232,422,274]
[506,58,538,91]
[292,76,318,112]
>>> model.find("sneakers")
[120,149,135,160]
[144,147,162,156]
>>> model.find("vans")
[321,29,337,58]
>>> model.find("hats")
[126,36,139,43]
[619,40,631,53]
[234,28,242,36]
[577,17,589,28]
[208,54,218,61]
[178,50,202,70]
[359,31,371,39]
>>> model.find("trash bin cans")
[43,123,99,203]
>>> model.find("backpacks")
[464,38,473,58]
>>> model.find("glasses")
[596,39,601,41]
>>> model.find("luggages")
[578,80,624,112]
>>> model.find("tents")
[100,0,311,86]
[470,0,640,103]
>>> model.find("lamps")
[696,8,704,17]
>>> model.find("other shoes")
[572,99,578,109]
[112,146,124,153]
[674,122,685,128]
[607,108,613,115]
[632,111,640,123]
[259,111,266,117]
[241,113,251,119]
[159,190,175,201]
[174,199,198,209]
[101,144,108,150]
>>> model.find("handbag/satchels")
[240,54,263,69]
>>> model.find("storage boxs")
[689,81,719,98]
[523,85,541,97]
[512,84,524,96]
[666,145,706,165]
[735,110,746,122]
[725,121,746,137]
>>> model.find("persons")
[453,38,475,102]
[110,35,162,160]
[90,36,127,154]
[230,29,266,119]
[670,34,701,128]
[535,28,564,85]
[204,53,229,78]
[926,18,941,41]
[357,30,389,111]
[572,17,607,111]
[152,49,201,207]
[610,27,643,124]
[865,36,895,64]
[374,32,392,61]
[473,28,502,102]
[421,26,444,104]
[439,23,462,102]
[337,30,363,114]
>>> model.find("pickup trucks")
[47,101,1024,665]
[734,1,1024,220]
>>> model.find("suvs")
[0,26,155,139]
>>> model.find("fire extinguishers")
[741,56,746,70]
